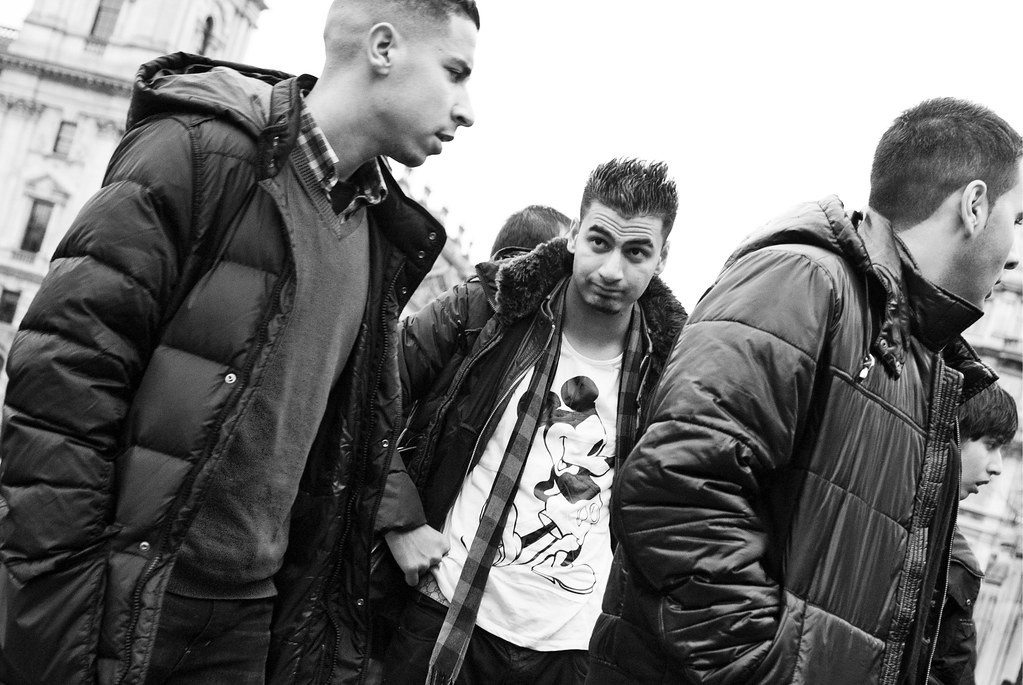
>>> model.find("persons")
[364,97,1023,685]
[0,0,481,685]
